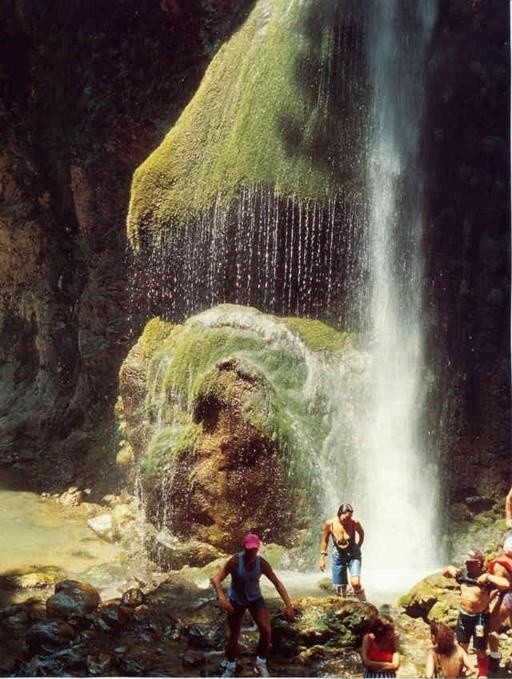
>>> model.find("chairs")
[475,655,498,666]
[255,657,269,676]
[221,660,237,677]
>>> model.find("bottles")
[242,533,260,550]
[466,548,486,562]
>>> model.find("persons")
[361,615,400,679]
[320,504,366,600]
[428,488,512,679]
[212,534,294,677]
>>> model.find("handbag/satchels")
[320,551,328,556]
